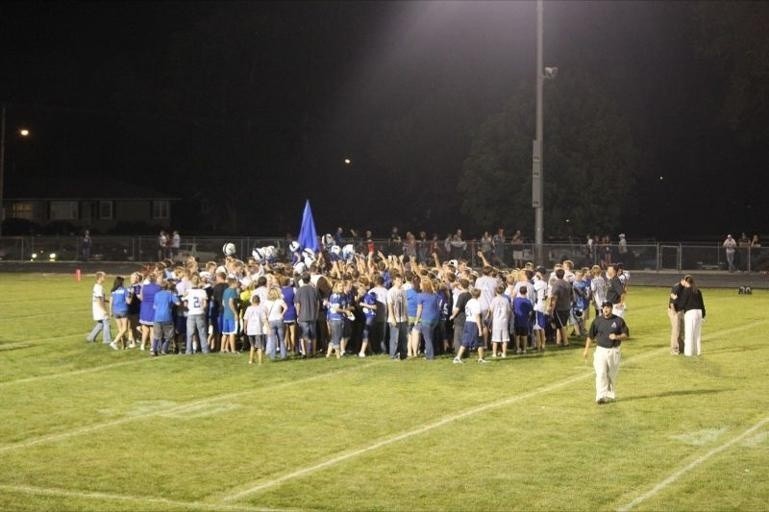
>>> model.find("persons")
[581,301,629,404]
[666,274,707,357]
[70,230,631,365]
[722,231,764,273]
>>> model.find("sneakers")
[478,353,507,362]
[109,342,168,356]
[454,356,463,364]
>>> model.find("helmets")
[322,234,352,258]
[222,240,236,256]
[573,307,584,318]
[252,245,276,261]
[289,241,301,252]
[296,247,316,273]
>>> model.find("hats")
[602,300,612,307]
[536,267,545,273]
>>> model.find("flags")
[293,198,319,251]
